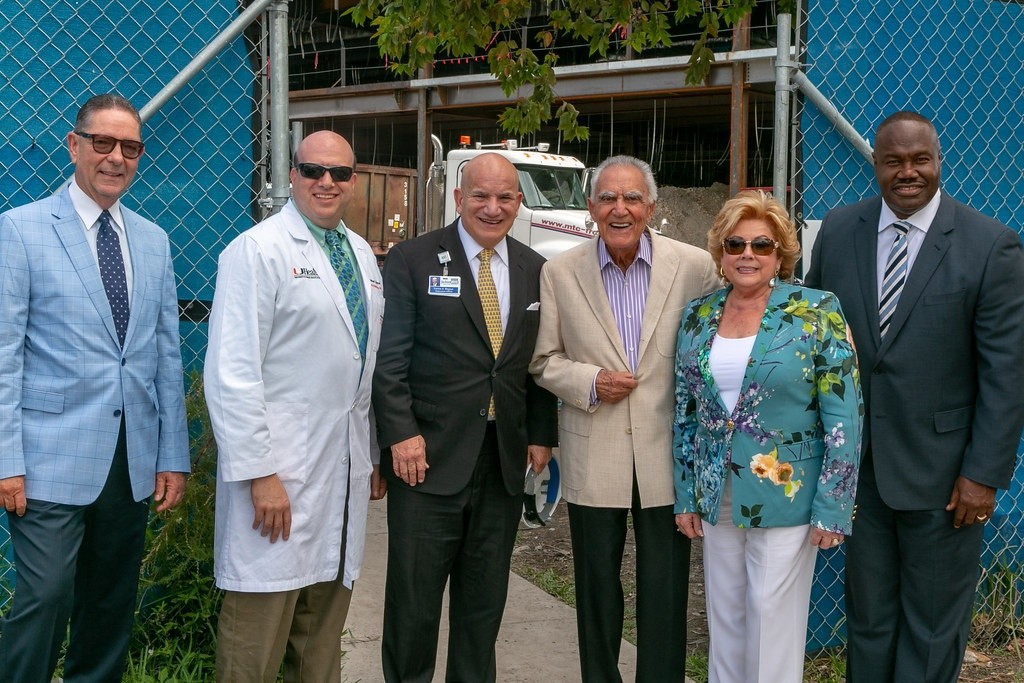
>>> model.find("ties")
[97,209,130,352]
[879,220,912,347]
[325,229,368,384]
[477,249,503,418]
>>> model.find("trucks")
[284,121,592,265]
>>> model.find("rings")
[832,539,838,543]
[976,514,987,521]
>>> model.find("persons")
[203,130,385,683]
[370,153,559,683]
[801,110,1024,683]
[528,156,725,683]
[670,189,865,683]
[0,94,191,683]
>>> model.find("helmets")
[521,453,563,528]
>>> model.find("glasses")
[77,132,145,159]
[721,236,780,256]
[296,163,354,182]
